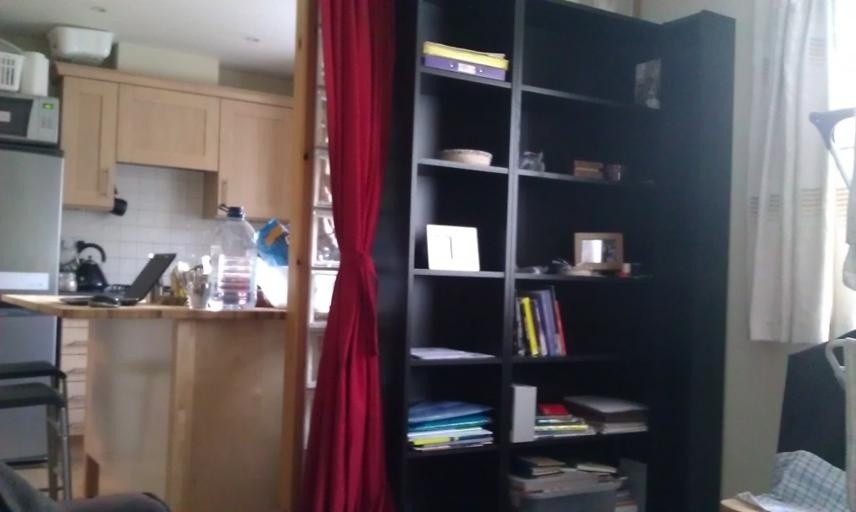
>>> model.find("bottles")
[57,265,79,293]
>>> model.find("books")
[424,55,506,81]
[532,395,650,436]
[408,399,495,451]
[508,454,639,512]
[512,283,567,356]
[421,35,510,69]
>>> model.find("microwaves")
[0,89,60,149]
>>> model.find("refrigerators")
[0,142,66,465]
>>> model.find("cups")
[184,282,213,309]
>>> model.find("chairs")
[0,462,172,511]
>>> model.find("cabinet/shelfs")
[204,99,307,222]
[373,0,661,512]
[118,84,219,170]
[56,74,117,209]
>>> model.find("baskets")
[1,52,26,92]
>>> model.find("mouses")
[88,294,120,308]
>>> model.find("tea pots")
[68,241,110,293]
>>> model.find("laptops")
[59,254,176,305]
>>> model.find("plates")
[440,146,494,167]
